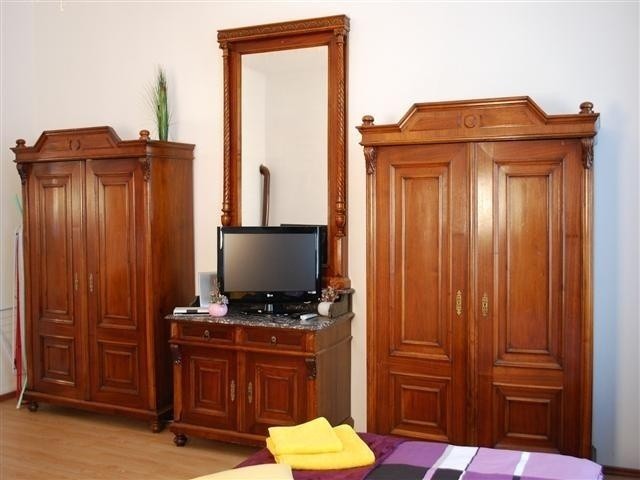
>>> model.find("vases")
[208,304,229,317]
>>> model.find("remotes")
[299,313,317,320]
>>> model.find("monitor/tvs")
[216,225,326,315]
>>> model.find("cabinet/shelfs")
[9,122,196,434]
[168,316,354,451]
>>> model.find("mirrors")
[216,13,351,289]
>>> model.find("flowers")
[208,290,230,306]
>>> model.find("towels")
[231,416,411,479]
